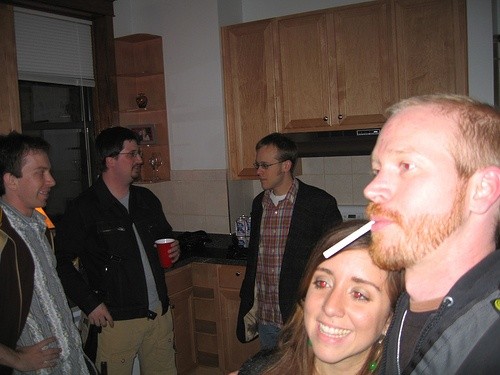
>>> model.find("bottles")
[234,214,251,248]
[136,93,148,108]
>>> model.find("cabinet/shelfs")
[222,0,467,180]
[114,33,170,185]
[165,263,262,375]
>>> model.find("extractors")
[280,128,380,158]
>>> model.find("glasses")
[253,160,284,170]
[118,150,143,158]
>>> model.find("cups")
[155,239,175,268]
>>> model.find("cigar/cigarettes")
[322,221,374,260]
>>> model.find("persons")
[54,130,182,375]
[237,220,405,375]
[0,129,91,375]
[230,94,500,374]
[236,131,344,350]
